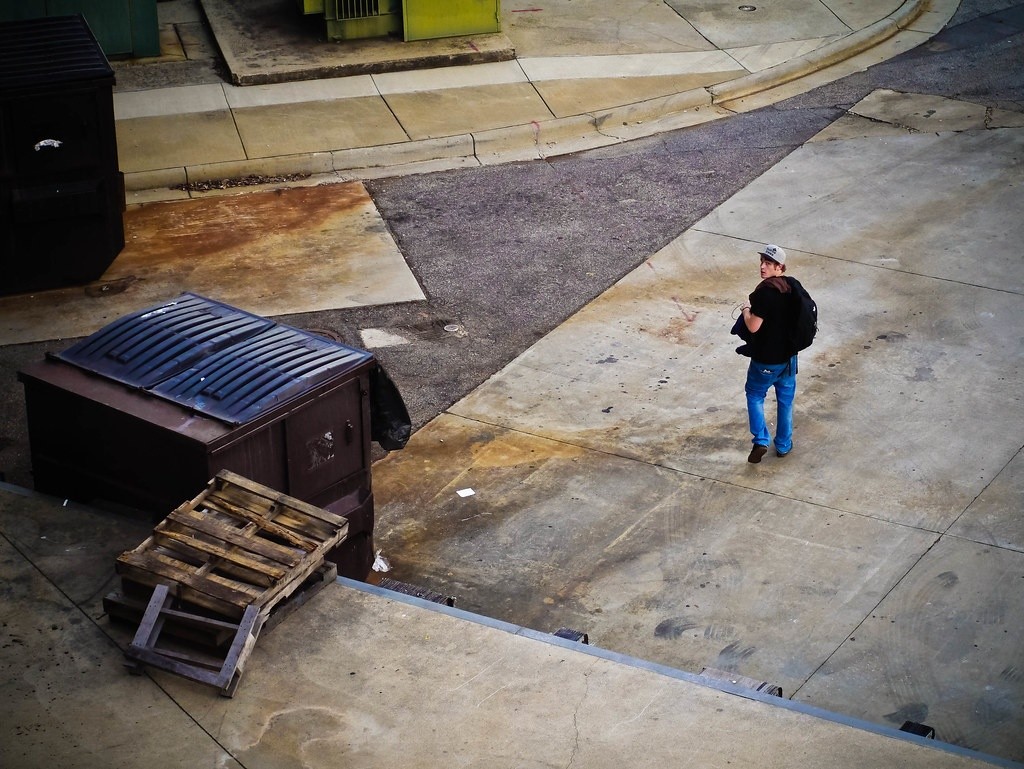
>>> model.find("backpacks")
[781,276,818,353]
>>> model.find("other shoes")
[777,439,793,457]
[748,443,767,463]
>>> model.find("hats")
[757,244,786,263]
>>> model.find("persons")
[729,243,803,464]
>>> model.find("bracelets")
[742,306,751,312]
